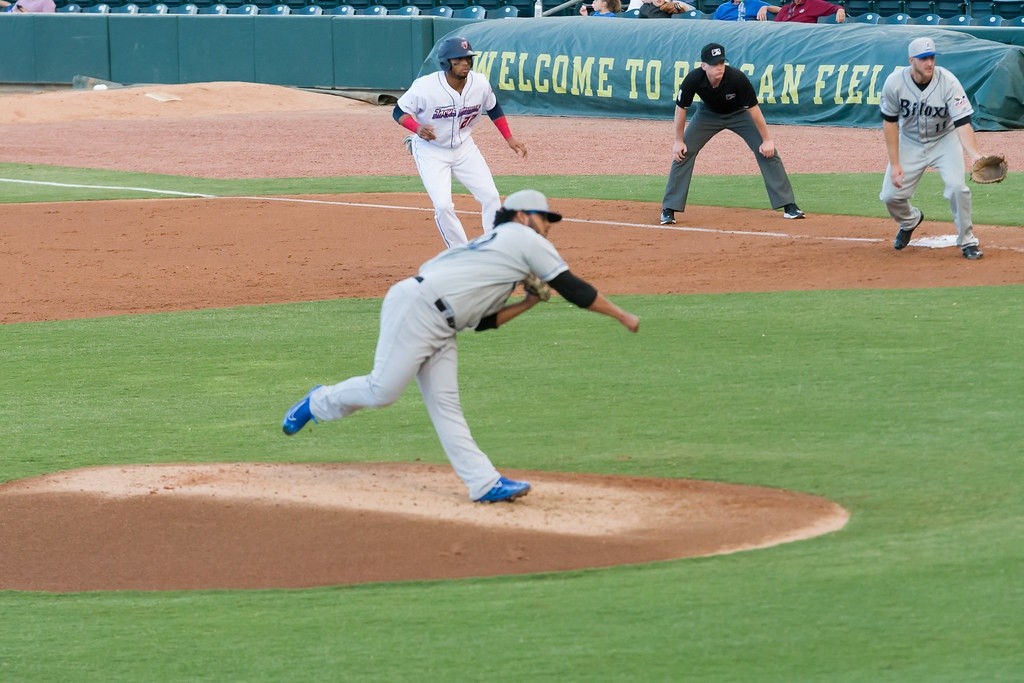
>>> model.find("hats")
[700,43,729,64]
[908,37,942,60]
[503,189,563,222]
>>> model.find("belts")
[414,276,455,328]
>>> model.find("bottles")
[535,0,542,17]
[738,0,746,21]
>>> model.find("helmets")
[438,37,477,72]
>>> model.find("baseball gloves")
[971,154,1008,184]
[524,274,551,302]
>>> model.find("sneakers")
[962,245,984,260]
[281,383,325,436]
[475,477,531,503]
[894,210,924,250]
[660,208,677,225]
[784,203,806,220]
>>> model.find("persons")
[878,37,1000,260]
[774,0,846,24]
[0,0,56,13]
[578,0,622,17]
[392,36,528,249]
[659,42,806,224]
[624,0,698,18]
[712,0,782,21]
[280,189,641,502]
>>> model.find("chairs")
[620,0,1024,28]
[55,0,519,20]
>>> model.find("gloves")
[403,135,413,155]
